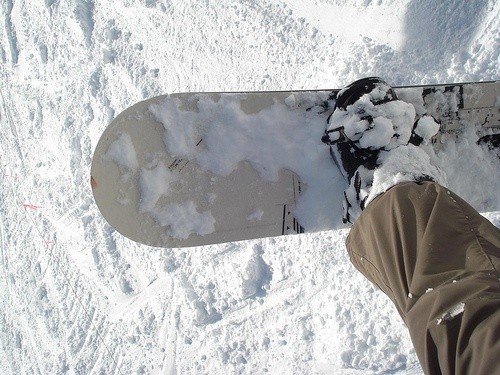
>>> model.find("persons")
[326,77,499,375]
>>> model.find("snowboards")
[91,80,500,248]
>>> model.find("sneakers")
[325,78,438,224]
